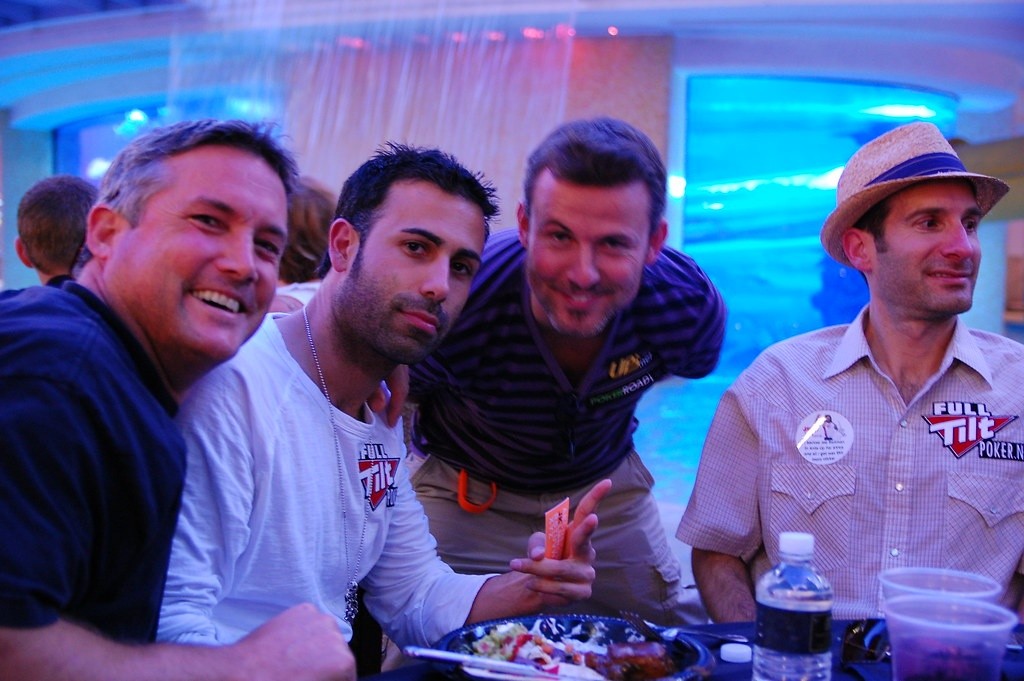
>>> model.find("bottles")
[751,533,834,681]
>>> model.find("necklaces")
[298,307,378,627]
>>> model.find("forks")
[618,608,749,651]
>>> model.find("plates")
[430,620,718,681]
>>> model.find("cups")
[882,594,1018,681]
[875,567,1005,613]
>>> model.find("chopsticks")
[400,645,571,679]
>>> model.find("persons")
[12,175,104,292]
[1,118,362,681]
[154,144,612,652]
[670,120,1023,622]
[377,114,732,674]
[266,170,342,317]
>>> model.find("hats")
[821,121,1012,267]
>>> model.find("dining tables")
[375,606,1024,681]
